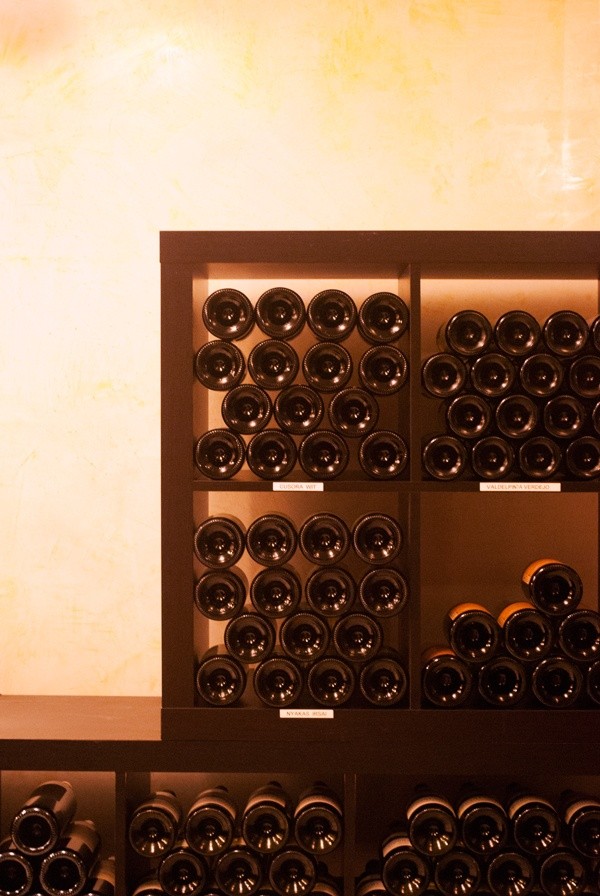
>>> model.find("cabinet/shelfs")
[1,231,600,896]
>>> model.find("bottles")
[195,287,408,480]
[420,558,600,710]
[422,310,600,480]
[0,780,600,896]
[193,511,406,707]
